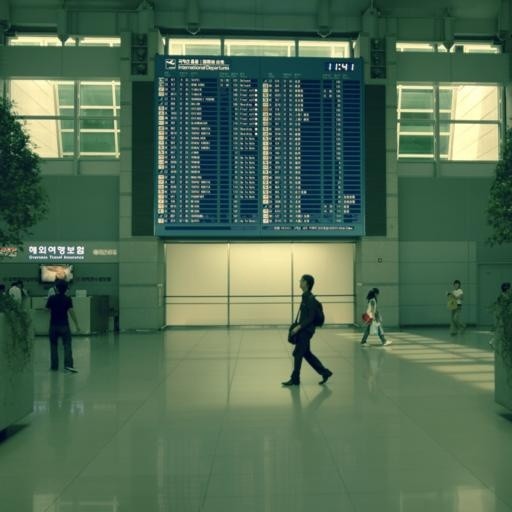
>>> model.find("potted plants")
[484,118,512,415]
[0,99,48,433]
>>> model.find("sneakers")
[360,343,370,347]
[384,340,392,345]
[64,365,79,373]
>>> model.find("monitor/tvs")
[39,263,74,286]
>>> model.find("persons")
[489,282,510,349]
[281,274,334,387]
[45,279,81,373]
[6,283,24,307]
[360,288,392,346]
[17,281,29,297]
[48,277,59,296]
[0,284,6,297]
[447,280,466,335]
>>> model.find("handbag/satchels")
[288,306,301,344]
[448,294,458,311]
[376,311,383,323]
[20,290,31,312]
[362,313,372,324]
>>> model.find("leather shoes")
[319,369,333,384]
[281,379,300,386]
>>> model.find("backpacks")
[312,298,324,327]
